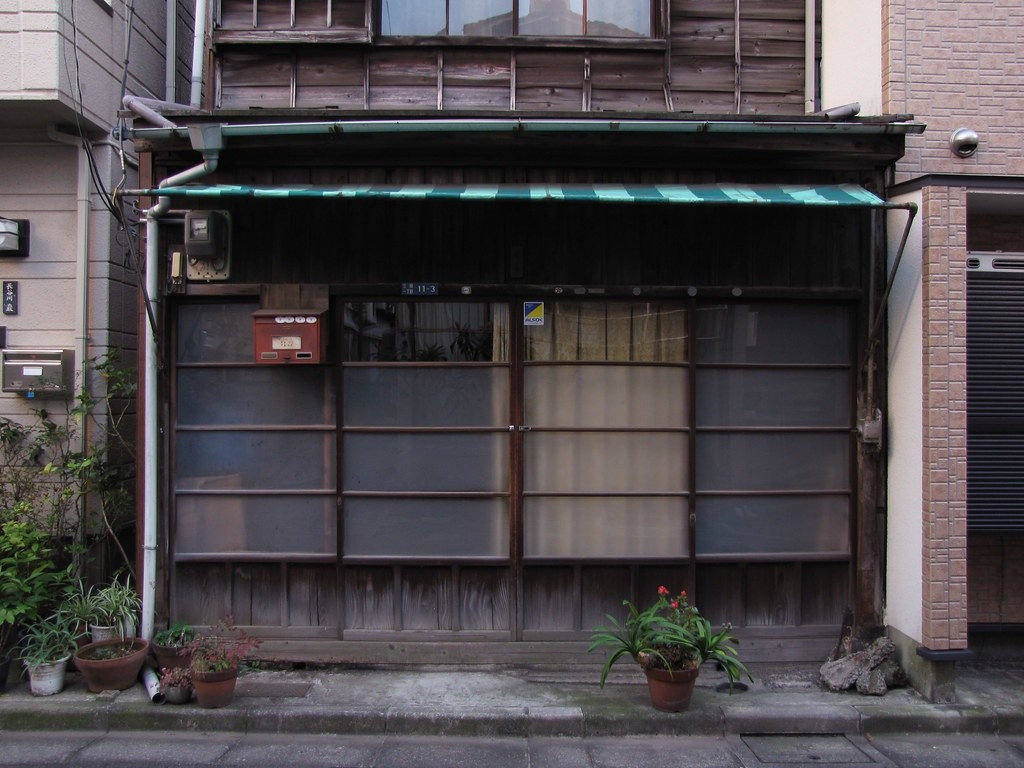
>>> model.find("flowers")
[587,586,756,694]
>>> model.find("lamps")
[0,216,30,259]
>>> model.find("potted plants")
[0,348,151,696]
[152,615,264,709]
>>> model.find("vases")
[641,663,699,713]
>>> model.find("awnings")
[114,174,884,207]
